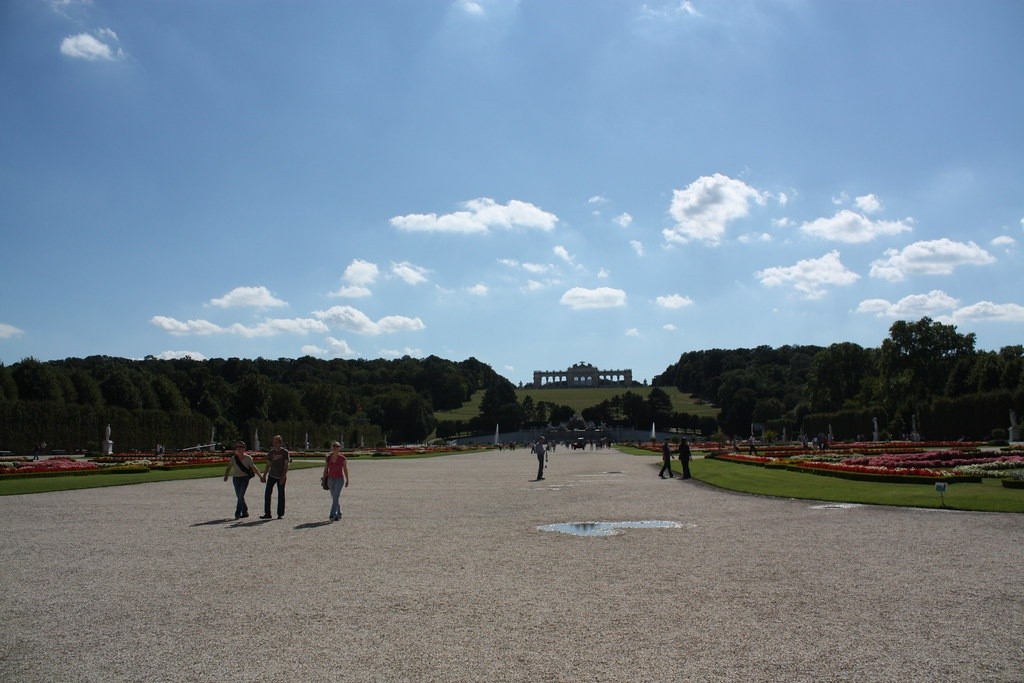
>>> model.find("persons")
[224,441,263,520]
[259,435,288,519]
[324,441,348,521]
[733,435,739,453]
[221,444,225,452]
[156,444,163,458]
[658,437,676,478]
[33,441,46,460]
[804,432,833,450]
[748,433,757,455]
[106,424,111,440]
[197,444,200,451]
[498,436,611,454]
[536,436,547,480]
[173,447,184,454]
[678,437,692,480]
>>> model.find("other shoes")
[659,474,664,477]
[682,476,691,479]
[239,512,249,518]
[335,514,342,521]
[329,515,335,521]
[235,513,240,519]
[277,515,283,519]
[259,514,272,519]
[670,476,675,478]
[537,478,545,480]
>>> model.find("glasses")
[236,446,245,448]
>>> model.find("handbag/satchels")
[321,475,329,490]
[248,468,255,479]
